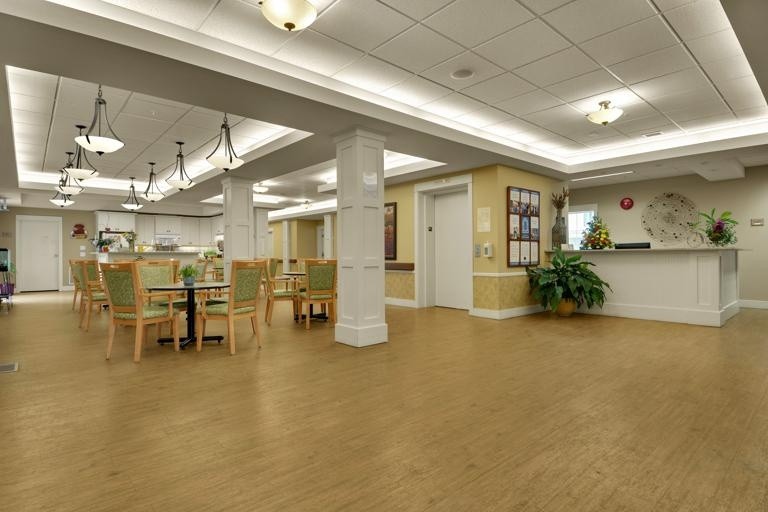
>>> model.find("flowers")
[123,231,137,242]
[687,208,738,246]
[95,238,113,248]
[579,214,613,249]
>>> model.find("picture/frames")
[384,202,398,260]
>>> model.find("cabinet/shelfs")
[94,211,136,239]
[135,214,223,246]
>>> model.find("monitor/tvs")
[614,242,651,249]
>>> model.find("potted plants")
[551,186,571,250]
[525,248,613,316]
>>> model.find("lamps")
[74,83,125,157]
[258,1,318,31]
[49,170,75,207]
[140,161,165,202]
[165,141,197,191]
[121,176,143,211]
[55,152,86,195]
[205,112,244,172]
[585,101,624,127]
[63,124,99,181]
[253,183,269,194]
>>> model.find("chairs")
[66,259,337,363]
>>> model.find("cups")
[561,243,574,251]
[138,246,143,253]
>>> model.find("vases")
[128,240,134,254]
[101,247,108,253]
[590,244,605,249]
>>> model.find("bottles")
[154,240,180,252]
[513,231,518,239]
